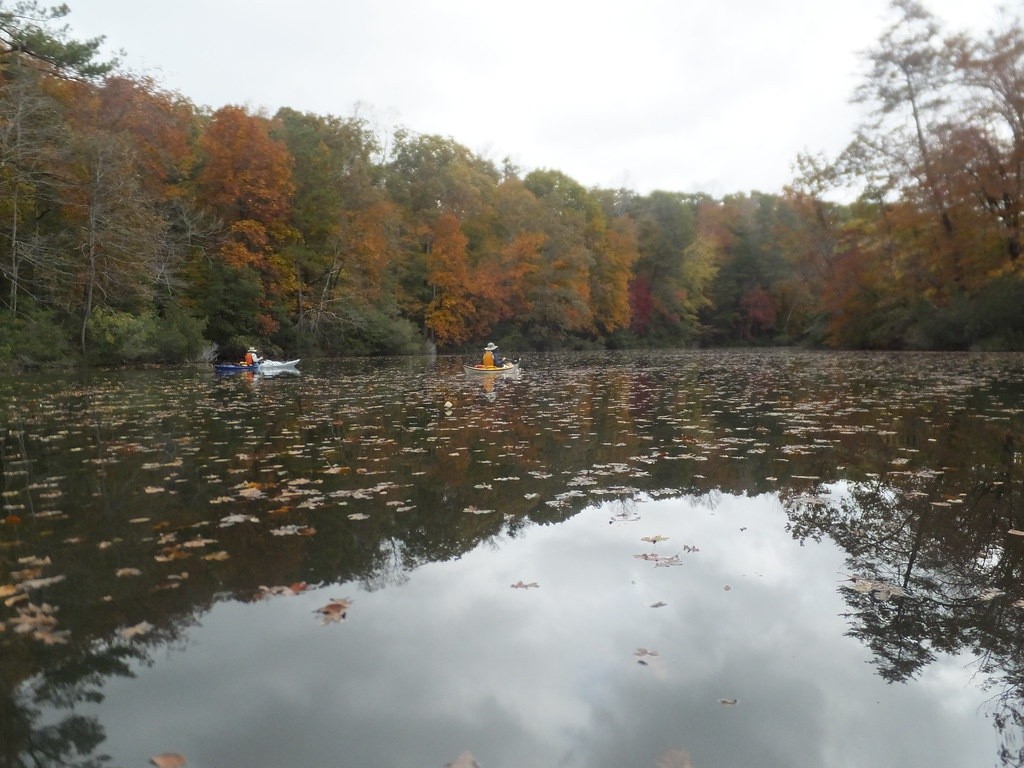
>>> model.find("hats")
[484,342,498,350]
[247,347,257,353]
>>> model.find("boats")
[462,358,524,377]
[258,359,300,370]
[260,368,301,379]
[213,358,264,376]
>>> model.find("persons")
[248,347,263,363]
[483,342,506,367]
